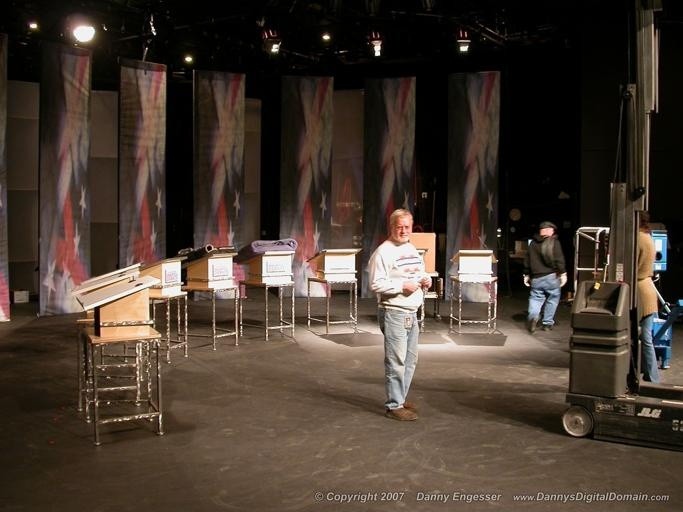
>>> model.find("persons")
[522,222,568,333]
[631,212,663,385]
[368,209,433,421]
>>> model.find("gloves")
[523,275,531,287]
[559,272,568,287]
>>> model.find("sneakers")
[529,318,536,333]
[404,401,417,409]
[542,325,553,332]
[384,408,417,421]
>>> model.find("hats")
[540,222,557,229]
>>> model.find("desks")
[447,275,500,335]
[177,285,241,350]
[149,290,188,364]
[305,276,359,335]
[377,269,443,333]
[81,318,164,448]
[238,277,295,343]
[76,314,151,410]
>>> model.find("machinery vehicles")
[560,1,681,450]
